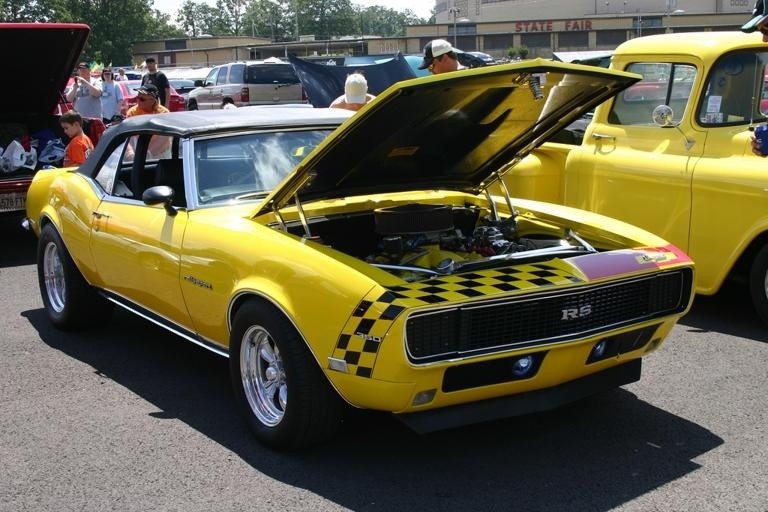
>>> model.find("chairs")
[157,159,184,199]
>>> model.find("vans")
[184,60,304,111]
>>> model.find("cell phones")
[75,77,77,84]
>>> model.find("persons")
[58,110,94,167]
[140,59,169,109]
[418,38,467,74]
[97,68,123,122]
[739,1,768,158]
[65,62,104,124]
[125,84,171,160]
[116,69,130,81]
[329,72,377,113]
[111,112,135,162]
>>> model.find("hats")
[417,39,454,70]
[103,69,112,74]
[78,62,89,69]
[145,58,156,63]
[133,83,159,99]
[344,72,368,105]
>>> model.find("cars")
[476,27,768,327]
[22,58,699,452]
